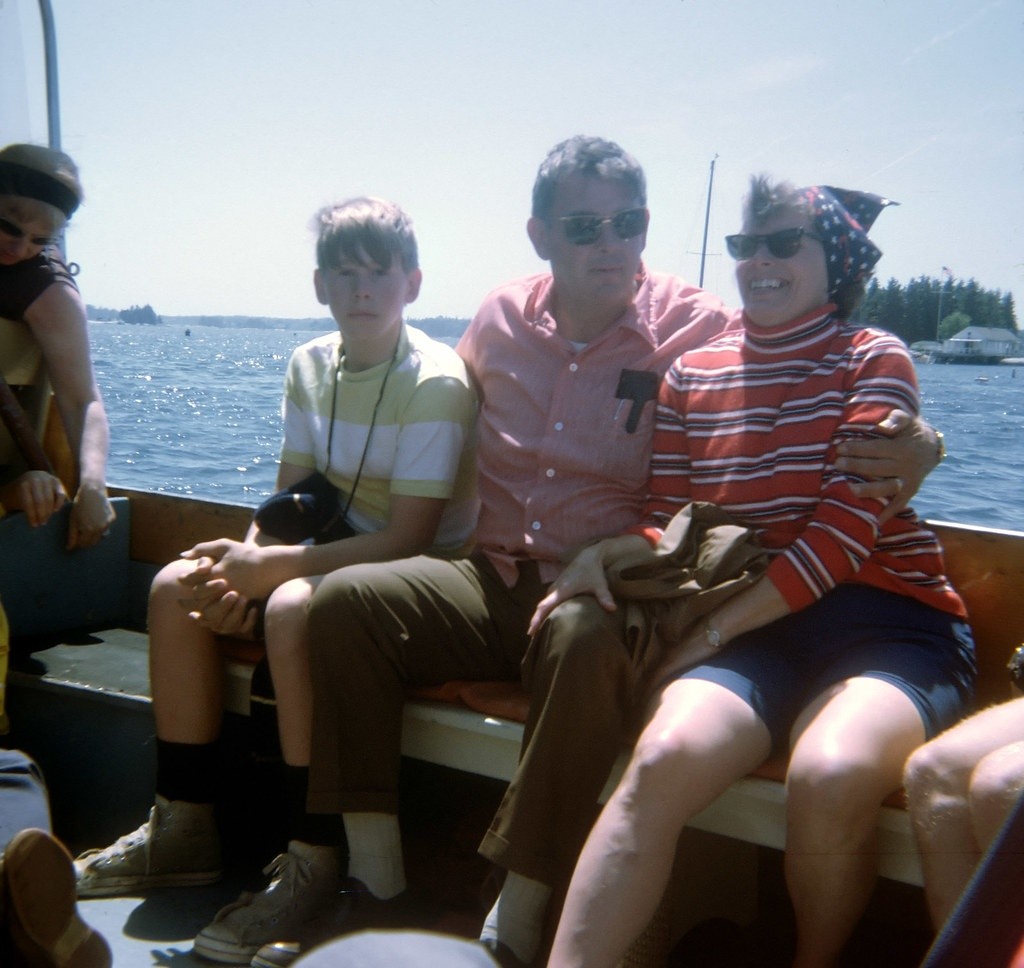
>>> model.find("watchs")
[703,620,722,652]
[923,422,945,464]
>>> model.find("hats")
[0,144,84,220]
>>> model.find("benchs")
[31,485,1024,885]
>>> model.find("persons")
[250,138,944,968]
[72,197,486,968]
[0,140,117,968]
[527,175,980,968]
[904,645,1024,938]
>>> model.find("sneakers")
[73,792,224,896]
[193,840,339,965]
[479,937,523,968]
[0,828,112,968]
[250,873,435,968]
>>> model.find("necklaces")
[322,322,402,519]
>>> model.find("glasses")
[725,225,826,259]
[550,206,646,245]
[0,218,57,246]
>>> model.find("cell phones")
[616,369,658,402]
